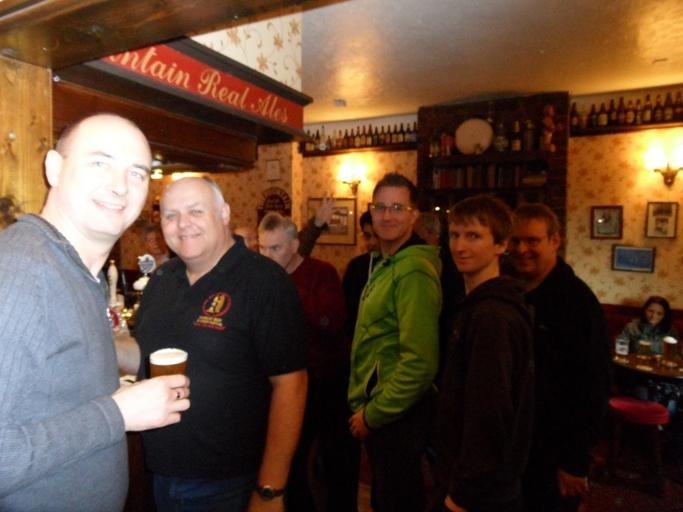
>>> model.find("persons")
[342,210,385,358]
[112,169,310,512]
[345,173,444,510]
[150,202,162,226]
[598,212,617,235]
[232,192,343,259]
[142,222,179,272]
[660,219,668,233]
[2,113,192,512]
[507,200,614,511]
[257,212,354,511]
[655,218,662,231]
[614,296,683,361]
[438,194,536,510]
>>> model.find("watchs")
[251,479,286,503]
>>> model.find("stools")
[608,397,668,473]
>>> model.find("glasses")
[510,233,553,247]
[368,204,413,217]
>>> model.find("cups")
[149,348,188,378]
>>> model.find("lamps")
[643,142,682,188]
[341,159,361,195]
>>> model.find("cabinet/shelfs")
[416,91,569,263]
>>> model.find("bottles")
[614,328,629,363]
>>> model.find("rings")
[174,389,181,400]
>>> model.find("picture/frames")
[645,202,678,238]
[306,197,356,245]
[611,244,655,273]
[590,206,621,239]
